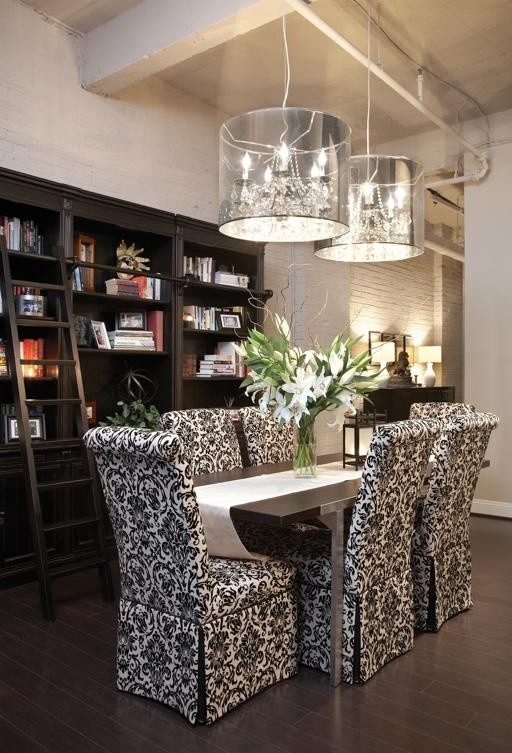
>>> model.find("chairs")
[259,418,442,686]
[238,406,334,528]
[343,412,500,632]
[82,426,299,727]
[160,409,317,550]
[404,399,475,420]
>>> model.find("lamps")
[371,342,395,381]
[417,346,442,387]
[311,1,427,264]
[218,0,352,243]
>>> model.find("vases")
[293,414,317,477]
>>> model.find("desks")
[192,454,495,688]
[362,386,455,424]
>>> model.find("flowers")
[229,263,432,478]
[230,262,401,424]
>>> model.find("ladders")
[0,235,115,621]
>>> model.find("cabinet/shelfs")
[175,215,274,408]
[1,173,182,588]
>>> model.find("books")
[182,255,211,281]
[106,330,155,350]
[0,337,44,377]
[3,216,41,252]
[195,342,249,378]
[106,276,161,296]
[183,305,245,332]
[8,285,43,316]
